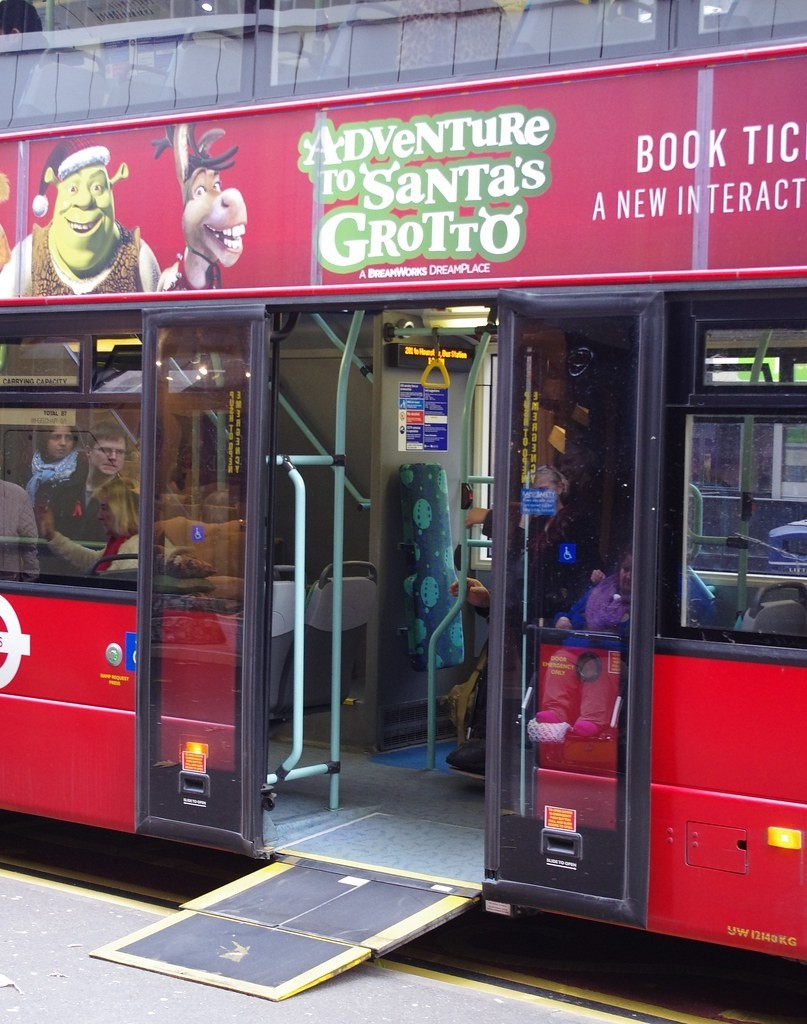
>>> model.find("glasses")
[94,448,125,456]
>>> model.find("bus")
[0,1,806,1004]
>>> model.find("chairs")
[739,582,807,638]
[0,0,806,139]
[279,561,378,721]
[266,563,298,722]
[87,553,140,583]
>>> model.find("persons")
[0,419,182,577]
[0,0,42,35]
[445,509,492,776]
[517,464,632,737]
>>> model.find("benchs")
[398,463,465,673]
